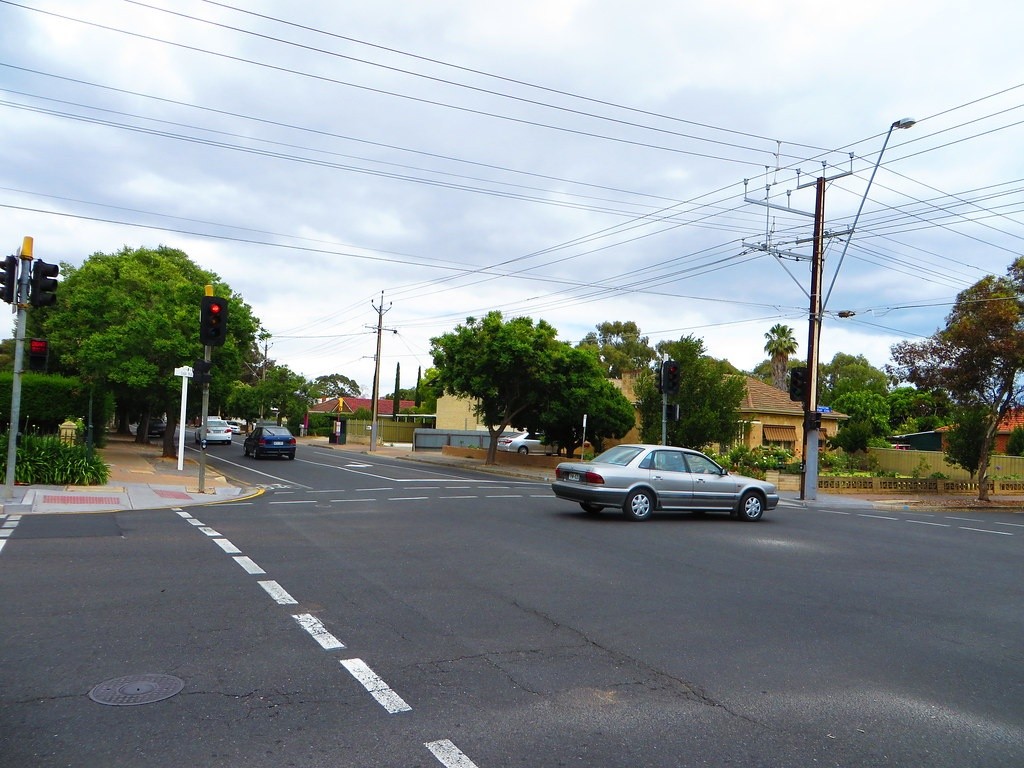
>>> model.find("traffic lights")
[29,259,59,307]
[201,296,227,346]
[790,367,809,401]
[0,255,16,303]
[193,359,213,383]
[654,361,680,395]
[28,337,49,371]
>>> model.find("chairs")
[662,455,674,469]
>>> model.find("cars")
[137,419,167,437]
[195,416,232,446]
[244,425,296,460]
[226,420,240,435]
[495,433,558,456]
[552,444,779,522]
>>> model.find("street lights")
[800,117,918,500]
